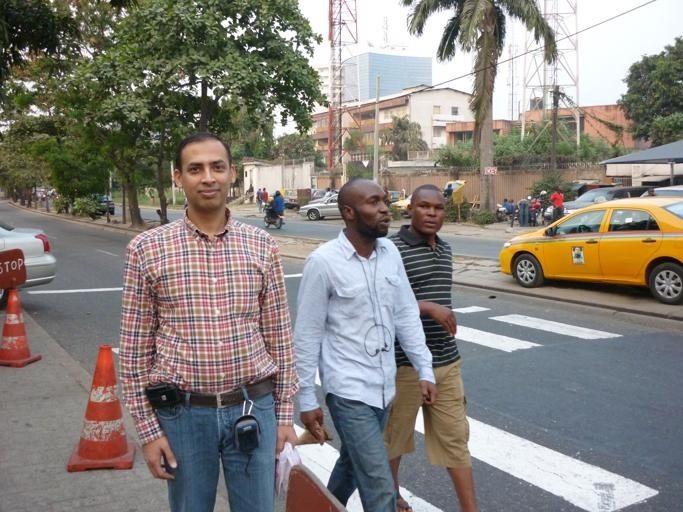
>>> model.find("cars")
[30,186,60,203]
[393,193,429,224]
[553,181,652,220]
[497,198,681,304]
[0,218,58,304]
[301,192,351,222]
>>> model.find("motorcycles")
[264,209,284,232]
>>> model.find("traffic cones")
[63,344,135,476]
[0,289,41,369]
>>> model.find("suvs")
[86,193,114,215]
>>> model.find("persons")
[499,185,566,233]
[291,177,437,512]
[382,187,479,512]
[443,184,454,204]
[117,134,300,512]
[256,187,287,224]
[324,187,331,198]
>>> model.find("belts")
[179,378,274,409]
